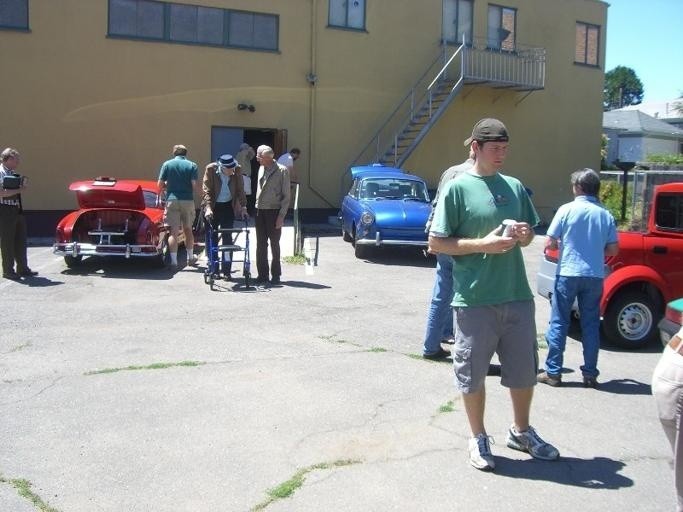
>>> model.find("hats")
[463,118,510,148]
[240,143,249,152]
[216,154,239,169]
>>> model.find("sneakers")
[213,273,220,279]
[583,376,596,387]
[224,274,231,281]
[169,264,177,272]
[187,254,199,266]
[271,277,280,285]
[507,425,560,462]
[536,371,562,387]
[467,433,497,470]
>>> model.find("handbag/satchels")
[3,173,25,216]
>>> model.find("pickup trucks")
[537,184,683,348]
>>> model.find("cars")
[656,297,683,347]
[53,176,194,273]
[338,163,436,265]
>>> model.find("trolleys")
[205,215,256,292]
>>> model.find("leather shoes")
[3,273,21,280]
[18,270,39,277]
[440,335,455,344]
[254,276,269,283]
[423,346,451,359]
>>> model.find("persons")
[536,169,619,387]
[422,140,475,357]
[0,148,37,279]
[156,144,301,283]
[651,328,683,511]
[428,118,560,470]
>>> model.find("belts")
[668,335,683,356]
[242,174,250,177]
